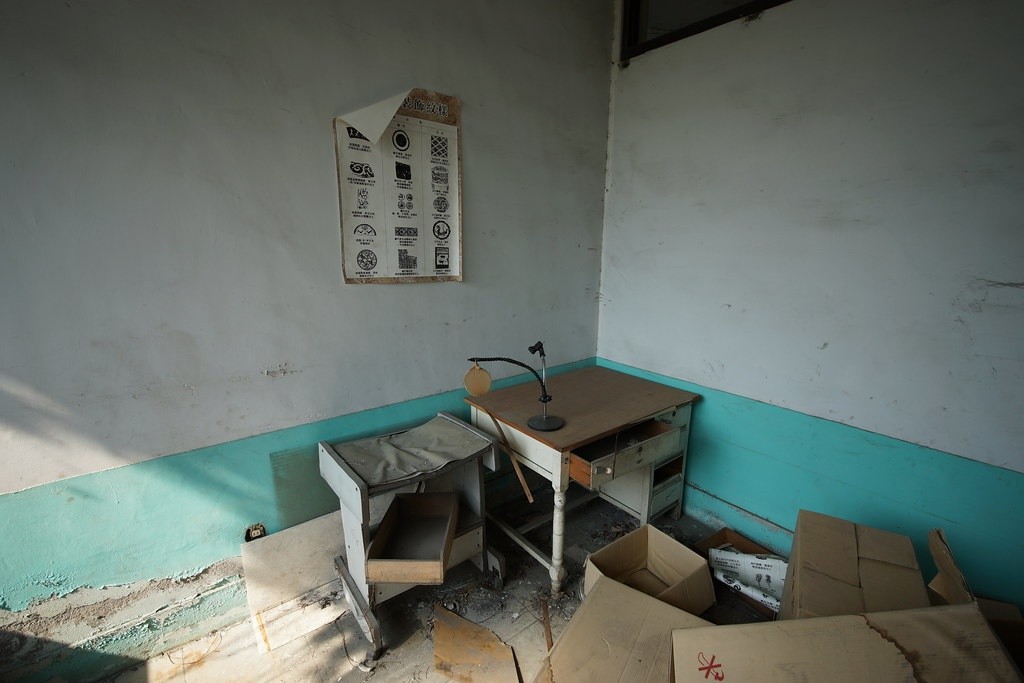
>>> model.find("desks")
[318,411,505,662]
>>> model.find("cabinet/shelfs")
[464,366,701,602]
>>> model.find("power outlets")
[243,524,267,542]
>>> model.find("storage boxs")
[777,510,931,620]
[532,574,715,683]
[583,523,717,617]
[692,527,785,618]
[668,603,1024,683]
[928,527,1024,646]
